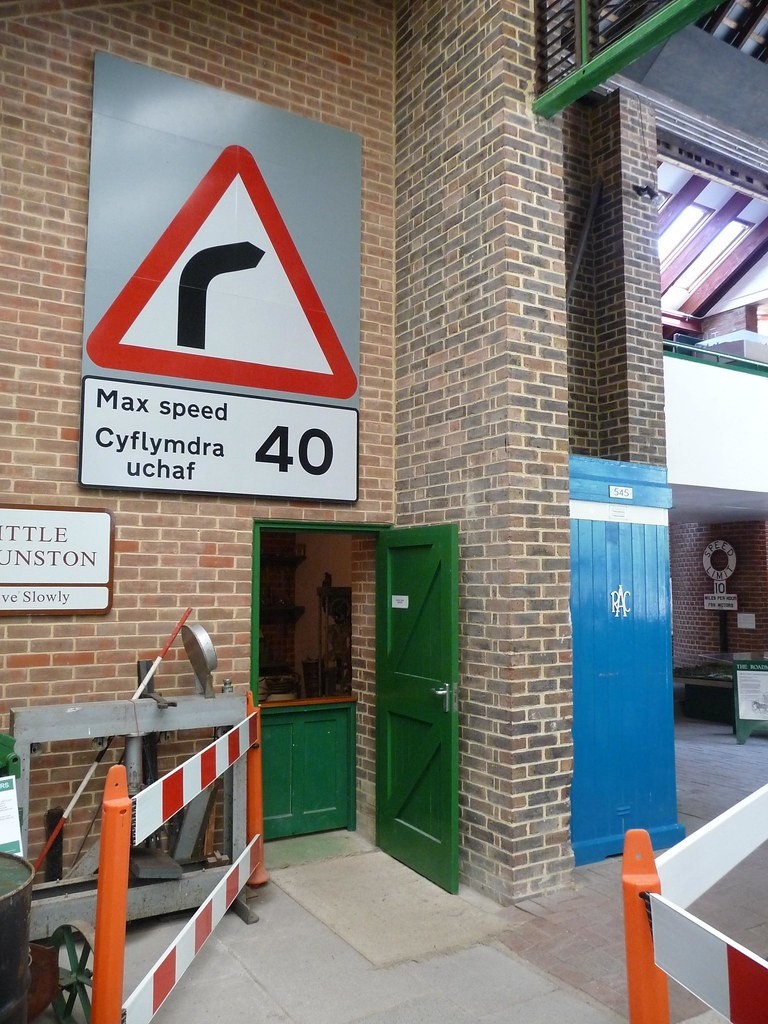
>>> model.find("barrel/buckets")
[0,850,37,1024]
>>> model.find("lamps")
[633,97,665,207]
